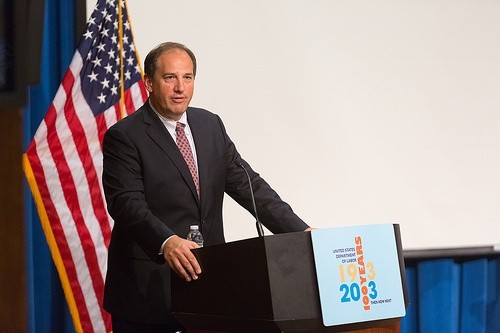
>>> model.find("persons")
[100,42,320,333]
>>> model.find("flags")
[23,0,150,333]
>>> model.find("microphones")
[236,160,264,237]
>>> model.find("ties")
[175,121,200,202]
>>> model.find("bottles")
[188,225,203,248]
